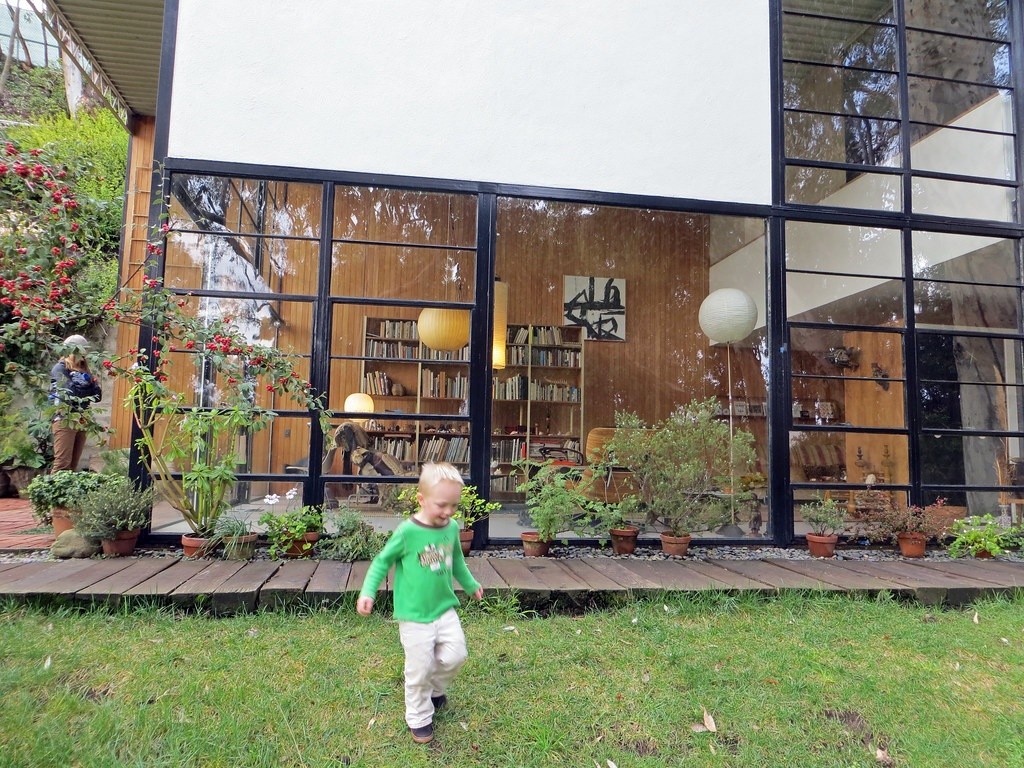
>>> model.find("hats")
[63,334,91,350]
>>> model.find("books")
[363,320,581,492]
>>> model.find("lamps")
[492,276,509,373]
[699,287,758,536]
[345,393,374,423]
[417,196,470,352]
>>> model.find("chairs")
[539,447,584,466]
[578,427,664,505]
[285,448,336,510]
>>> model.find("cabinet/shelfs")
[359,316,584,500]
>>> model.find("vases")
[899,531,928,556]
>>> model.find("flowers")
[849,485,1024,558]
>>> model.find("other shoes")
[431,695,446,707]
[412,725,433,743]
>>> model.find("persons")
[51,335,102,475]
[357,463,484,743]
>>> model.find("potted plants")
[259,506,325,560]
[127,362,269,559]
[18,471,115,539]
[194,510,256,559]
[66,475,162,556]
[396,481,501,556]
[588,439,652,553]
[512,456,606,557]
[800,489,844,557]
[614,397,758,553]
